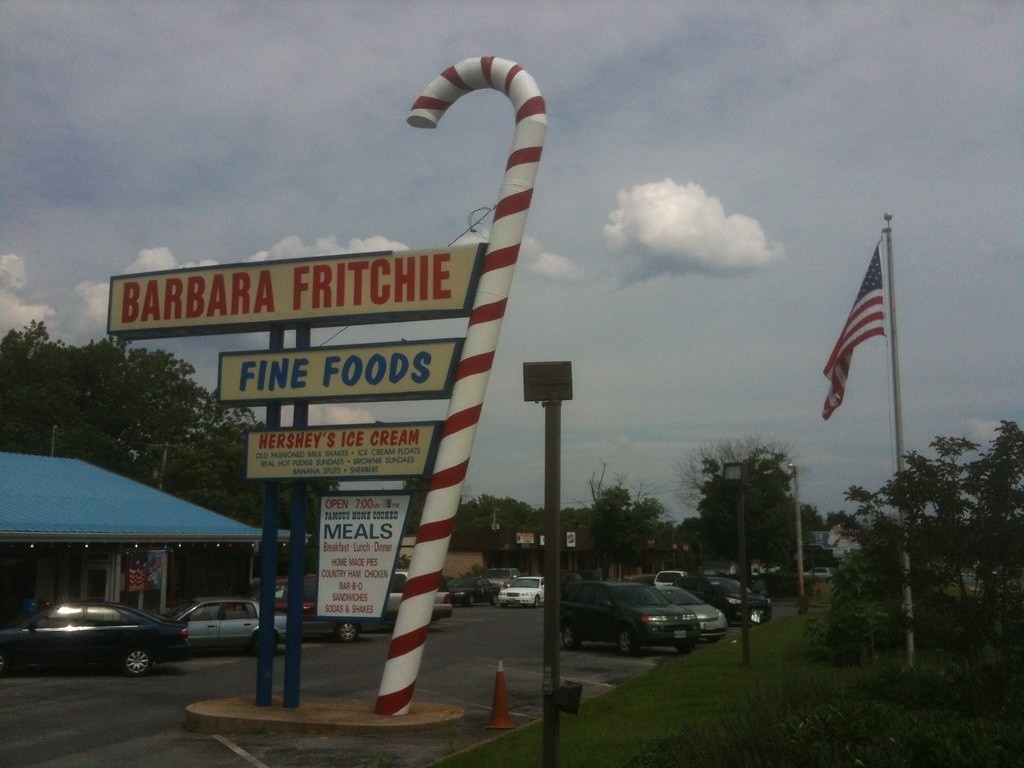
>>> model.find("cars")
[224,574,362,643]
[498,576,545,609]
[446,575,502,607]
[168,597,286,656]
[652,585,727,644]
[0,598,192,678]
[672,574,773,624]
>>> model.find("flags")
[820,242,886,422]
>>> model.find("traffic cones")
[479,660,518,729]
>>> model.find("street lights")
[788,463,806,616]
[523,360,583,768]
[720,461,751,664]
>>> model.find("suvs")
[384,568,453,622]
[559,580,702,658]
[484,567,520,593]
[654,570,690,588]
